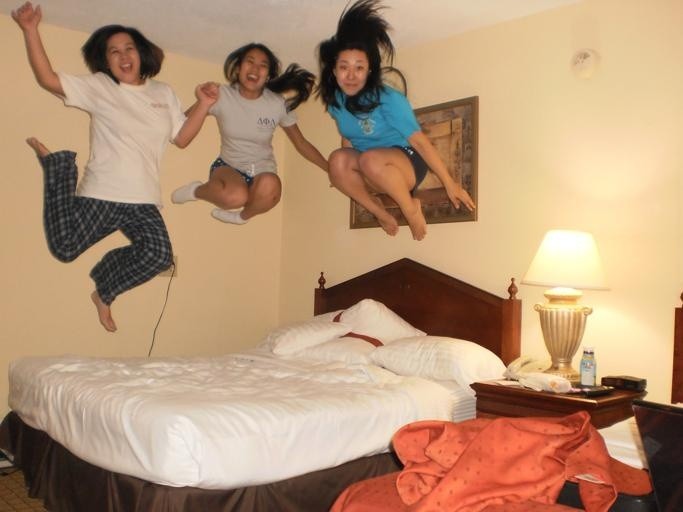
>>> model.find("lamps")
[522,229,616,385]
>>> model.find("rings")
[465,204,468,206]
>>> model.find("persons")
[9,2,218,334]
[170,43,335,224]
[312,0,476,241]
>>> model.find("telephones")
[503,354,552,380]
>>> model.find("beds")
[8,258,522,512]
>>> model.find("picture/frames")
[349,94,480,231]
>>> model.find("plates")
[571,385,615,396]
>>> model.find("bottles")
[579,350,596,391]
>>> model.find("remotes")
[587,386,615,395]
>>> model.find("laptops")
[632,399,683,512]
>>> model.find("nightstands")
[469,378,648,437]
